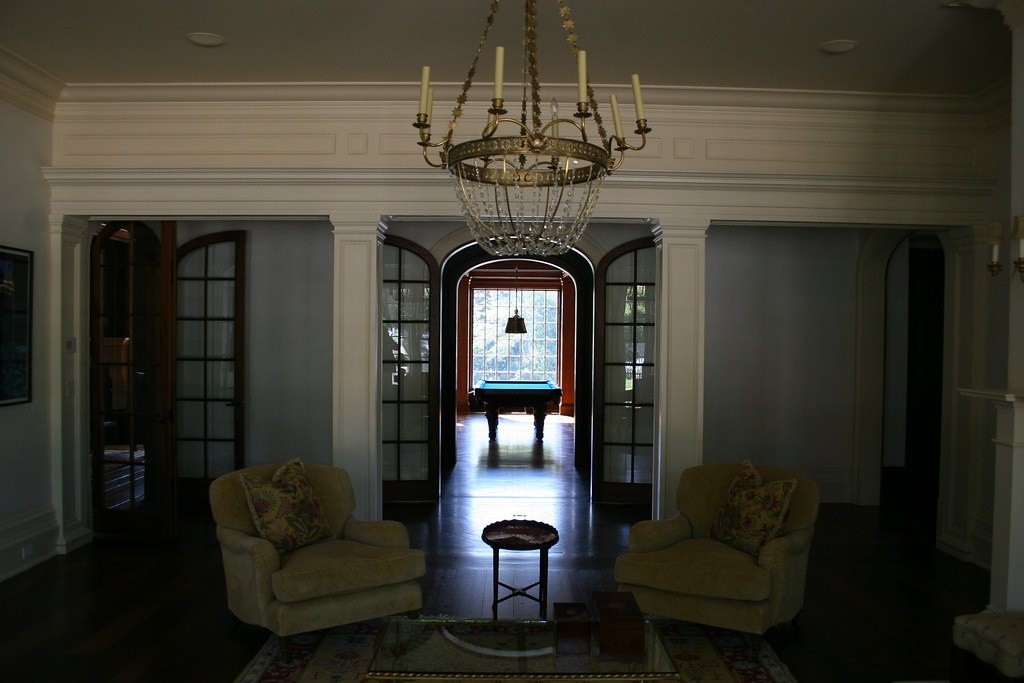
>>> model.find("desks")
[478,379,562,443]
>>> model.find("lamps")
[987,215,1024,286]
[411,0,652,258]
[503,260,529,333]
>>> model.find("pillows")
[704,456,800,557]
[237,457,336,556]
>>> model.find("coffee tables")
[363,615,682,683]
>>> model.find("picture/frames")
[0,245,34,404]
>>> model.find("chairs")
[211,465,427,661]
[614,459,820,664]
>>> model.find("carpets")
[229,607,800,683]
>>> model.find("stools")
[483,519,559,619]
